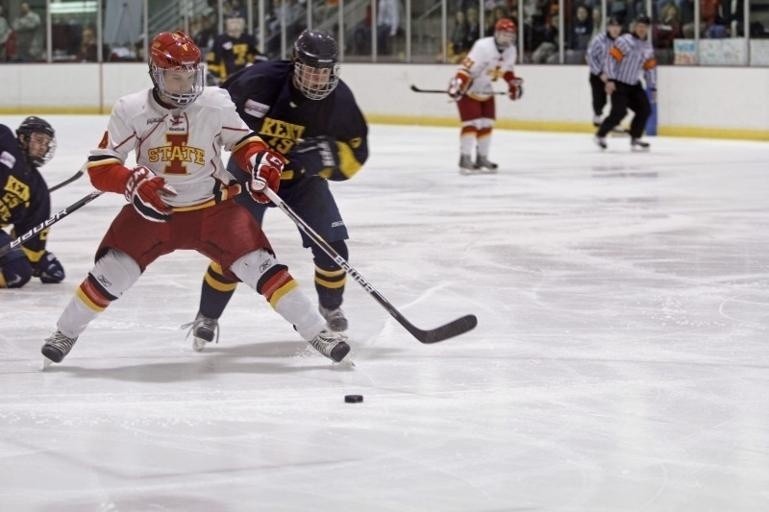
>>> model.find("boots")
[477,155,497,169]
[460,155,479,170]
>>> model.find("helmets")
[494,18,517,49]
[16,116,57,167]
[148,31,206,108]
[293,30,342,102]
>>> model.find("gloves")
[509,78,523,100]
[39,253,65,283]
[122,166,176,224]
[283,136,336,178]
[449,71,471,99]
[243,149,286,206]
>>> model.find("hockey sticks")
[263,186,477,344]
[48,160,88,192]
[410,85,507,96]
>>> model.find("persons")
[586,18,630,133]
[448,19,523,174]
[0,1,41,61]
[192,2,325,51]
[182,30,369,353]
[596,17,658,153]
[439,0,600,66]
[333,1,400,55]
[42,31,357,373]
[0,116,65,289]
[613,0,763,65]
[205,11,267,84]
[75,29,144,62]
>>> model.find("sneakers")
[592,115,649,148]
[318,304,347,331]
[41,329,78,362]
[308,325,350,362]
[192,311,216,342]
